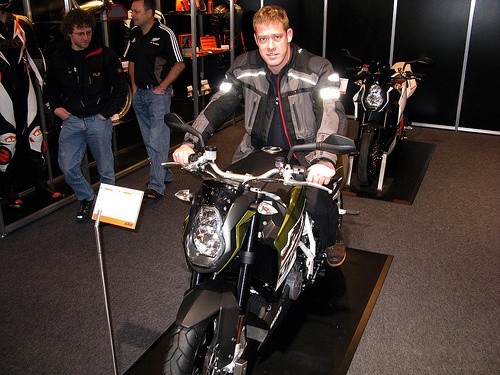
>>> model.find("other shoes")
[143,189,162,202]
[146,181,173,187]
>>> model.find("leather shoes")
[325,226,346,268]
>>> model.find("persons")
[173,5,347,268]
[42,9,131,223]
[122,1,187,203]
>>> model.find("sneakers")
[76,194,96,224]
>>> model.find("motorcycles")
[150,110,359,375]
[337,49,434,196]
